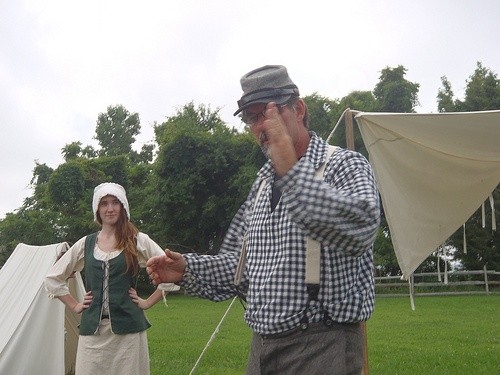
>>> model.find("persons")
[46,182,180,375]
[146,65,380,375]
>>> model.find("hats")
[92,182,131,225]
[233,65,299,116]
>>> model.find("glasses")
[241,103,288,124]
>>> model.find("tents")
[0,242,86,375]
